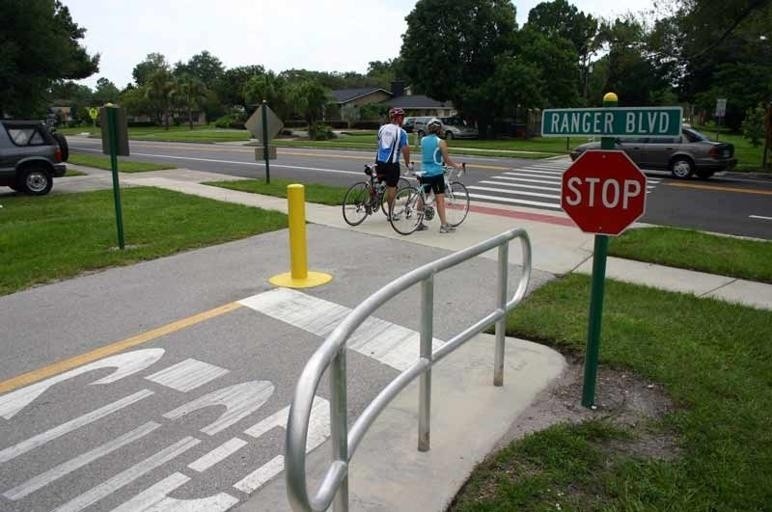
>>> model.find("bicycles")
[344,159,415,227]
[387,162,473,236]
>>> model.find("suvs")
[0,119,68,198]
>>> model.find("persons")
[368,107,463,233]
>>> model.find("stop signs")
[562,149,647,236]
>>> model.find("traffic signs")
[540,106,686,138]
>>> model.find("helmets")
[389,107,405,123]
[428,119,443,133]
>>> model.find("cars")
[401,117,480,139]
[568,129,739,180]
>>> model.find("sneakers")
[414,223,429,231]
[387,213,400,221]
[440,224,457,234]
